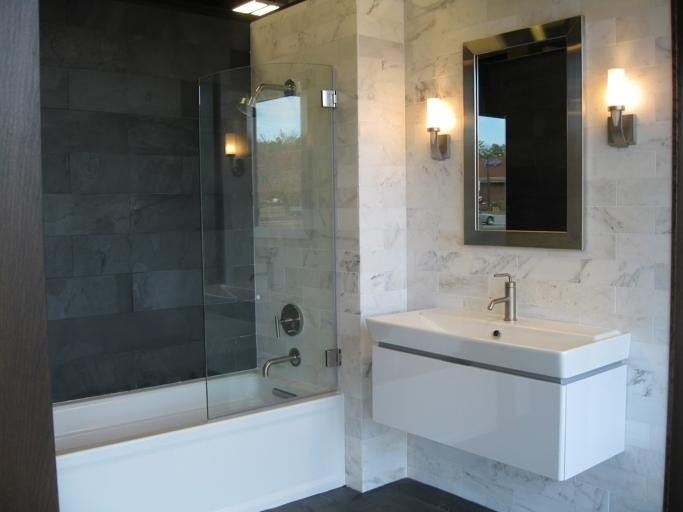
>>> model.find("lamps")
[607,68,636,147]
[225,126,244,178]
[427,97,450,161]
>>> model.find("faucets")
[262,356,297,377]
[489,273,518,321]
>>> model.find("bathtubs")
[53,368,343,512]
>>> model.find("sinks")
[365,309,629,480]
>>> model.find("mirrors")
[463,15,585,250]
